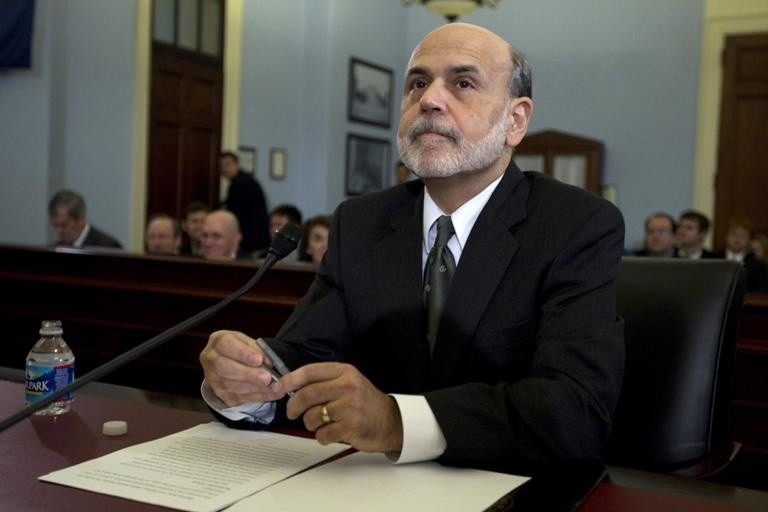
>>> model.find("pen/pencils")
[262,362,296,398]
[253,338,291,377]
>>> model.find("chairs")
[619,253,747,478]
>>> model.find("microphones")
[0,221,302,433]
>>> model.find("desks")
[2,378,767,512]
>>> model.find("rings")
[320,403,331,424]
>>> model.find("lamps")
[396,0,501,22]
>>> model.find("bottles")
[24,319,76,416]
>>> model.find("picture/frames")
[344,132,390,195]
[268,147,289,179]
[346,58,394,129]
[239,145,256,178]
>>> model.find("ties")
[422,216,457,354]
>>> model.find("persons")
[633,212,768,291]
[215,151,271,260]
[40,185,126,250]
[150,205,330,265]
[195,19,628,486]
